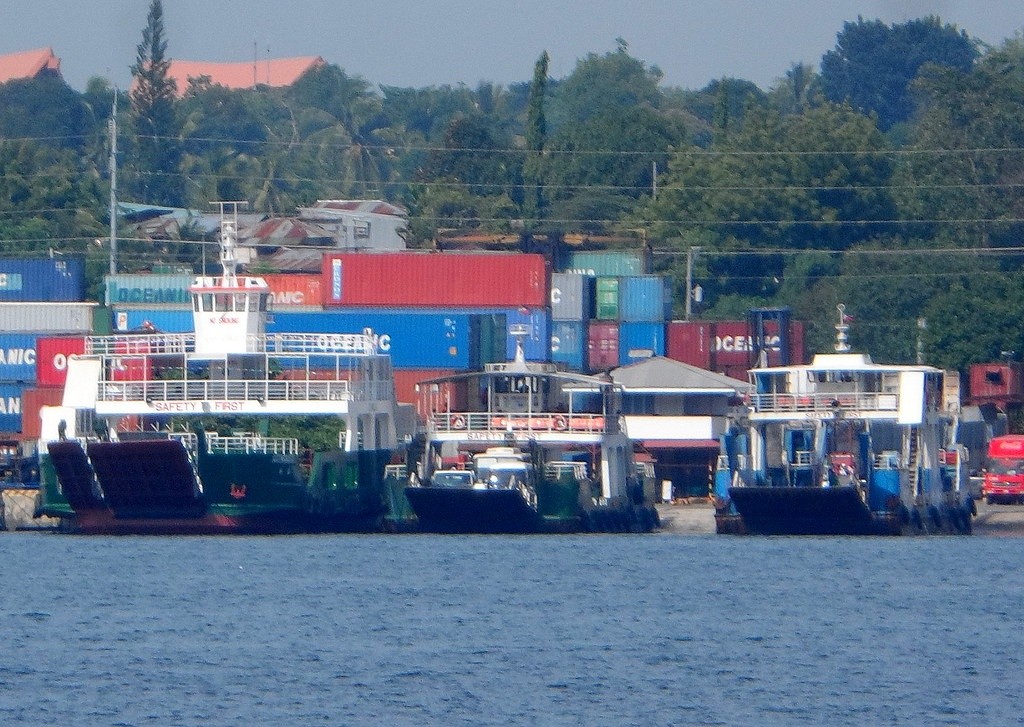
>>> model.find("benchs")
[821,397,860,410]
[778,397,809,412]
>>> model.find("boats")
[384,325,662,534]
[36,200,417,537]
[706,303,977,537]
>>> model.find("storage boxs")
[0,249,803,439]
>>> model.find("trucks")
[982,434,1024,503]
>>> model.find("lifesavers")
[552,415,567,431]
[451,415,465,429]
[291,384,302,395]
